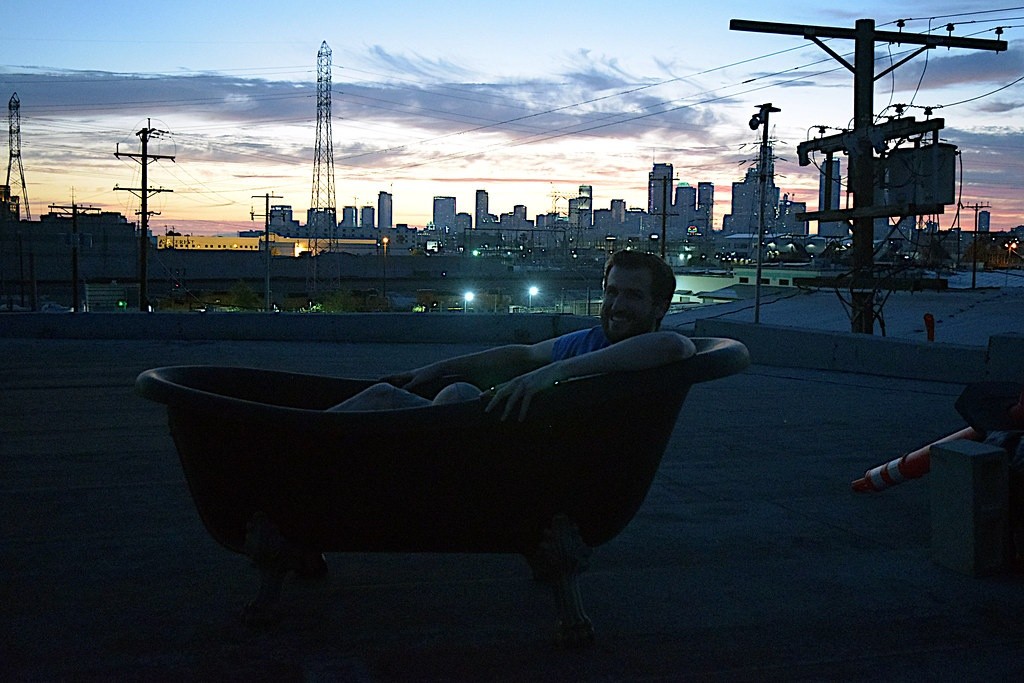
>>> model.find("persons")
[324,250,696,422]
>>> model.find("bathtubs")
[133,335,751,557]
